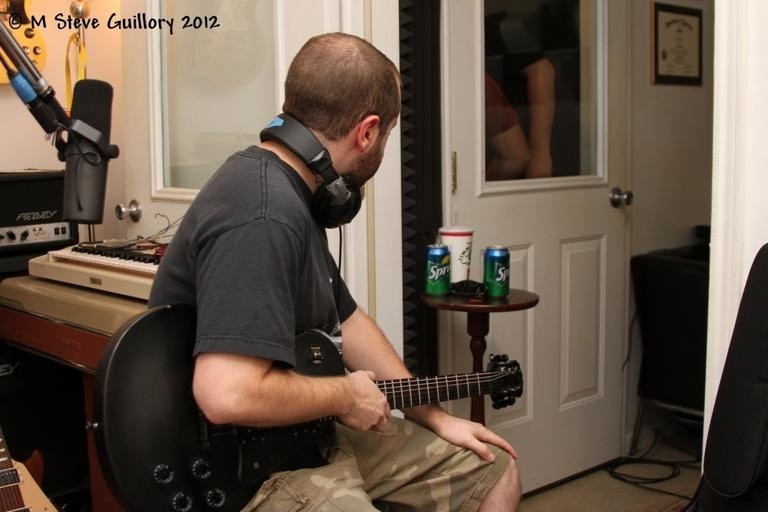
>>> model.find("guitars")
[94,305,523,511]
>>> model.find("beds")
[421,280,542,430]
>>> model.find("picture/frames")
[648,1,704,87]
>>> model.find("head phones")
[260,113,361,229]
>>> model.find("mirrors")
[147,0,285,205]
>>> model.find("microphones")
[62,79,113,225]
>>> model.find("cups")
[439,225,475,282]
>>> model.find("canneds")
[424,243,451,297]
[483,245,510,298]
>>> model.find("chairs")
[625,225,709,458]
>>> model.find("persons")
[481,67,530,178]
[146,31,525,511]
[522,15,584,176]
